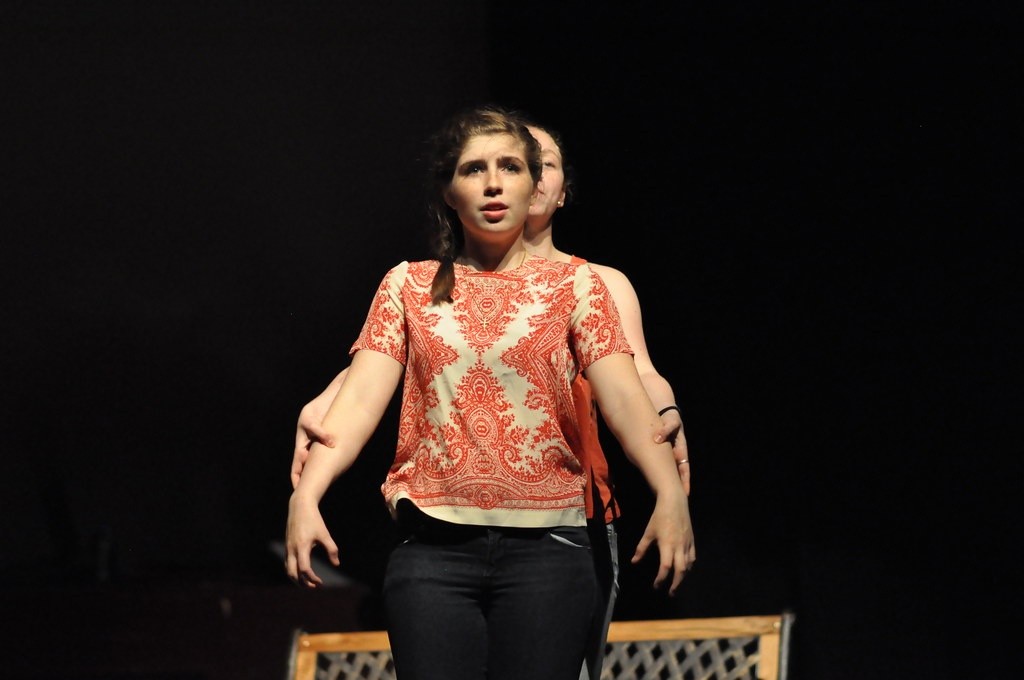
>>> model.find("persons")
[287,107,696,680]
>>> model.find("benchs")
[287,613,796,680]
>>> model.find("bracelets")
[658,405,681,415]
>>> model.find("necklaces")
[463,245,526,268]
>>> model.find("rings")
[677,459,688,467]
